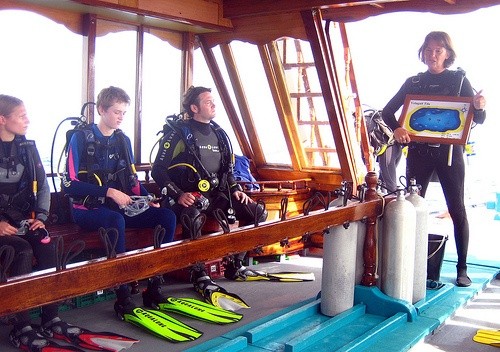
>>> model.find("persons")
[151,85,315,310]
[381,31,486,287]
[0,94,140,352]
[61,86,243,343]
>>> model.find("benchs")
[1,162,242,272]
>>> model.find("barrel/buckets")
[428,234,450,281]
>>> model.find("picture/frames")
[396,94,474,146]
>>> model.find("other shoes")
[456,276,472,287]
[141,288,172,311]
[224,264,258,281]
[9,319,83,352]
[190,269,224,301]
[113,298,144,325]
